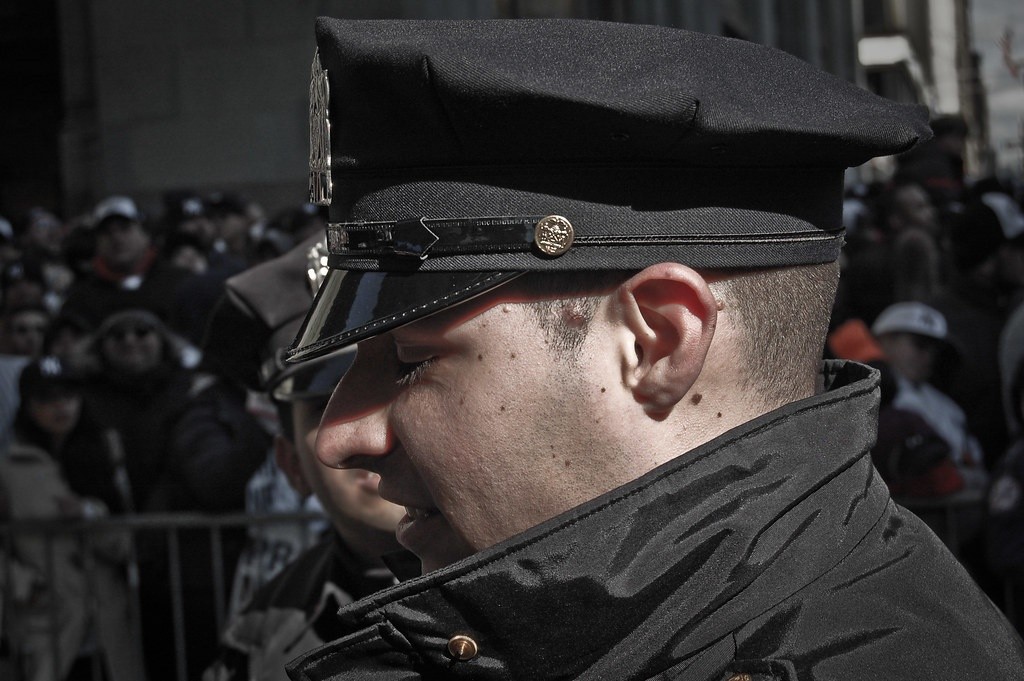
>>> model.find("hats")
[279,18,937,365]
[870,300,947,340]
[90,196,138,227]
[205,227,359,404]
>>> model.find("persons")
[0,114,1024,681]
[281,15,1024,681]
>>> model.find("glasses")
[102,322,151,344]
